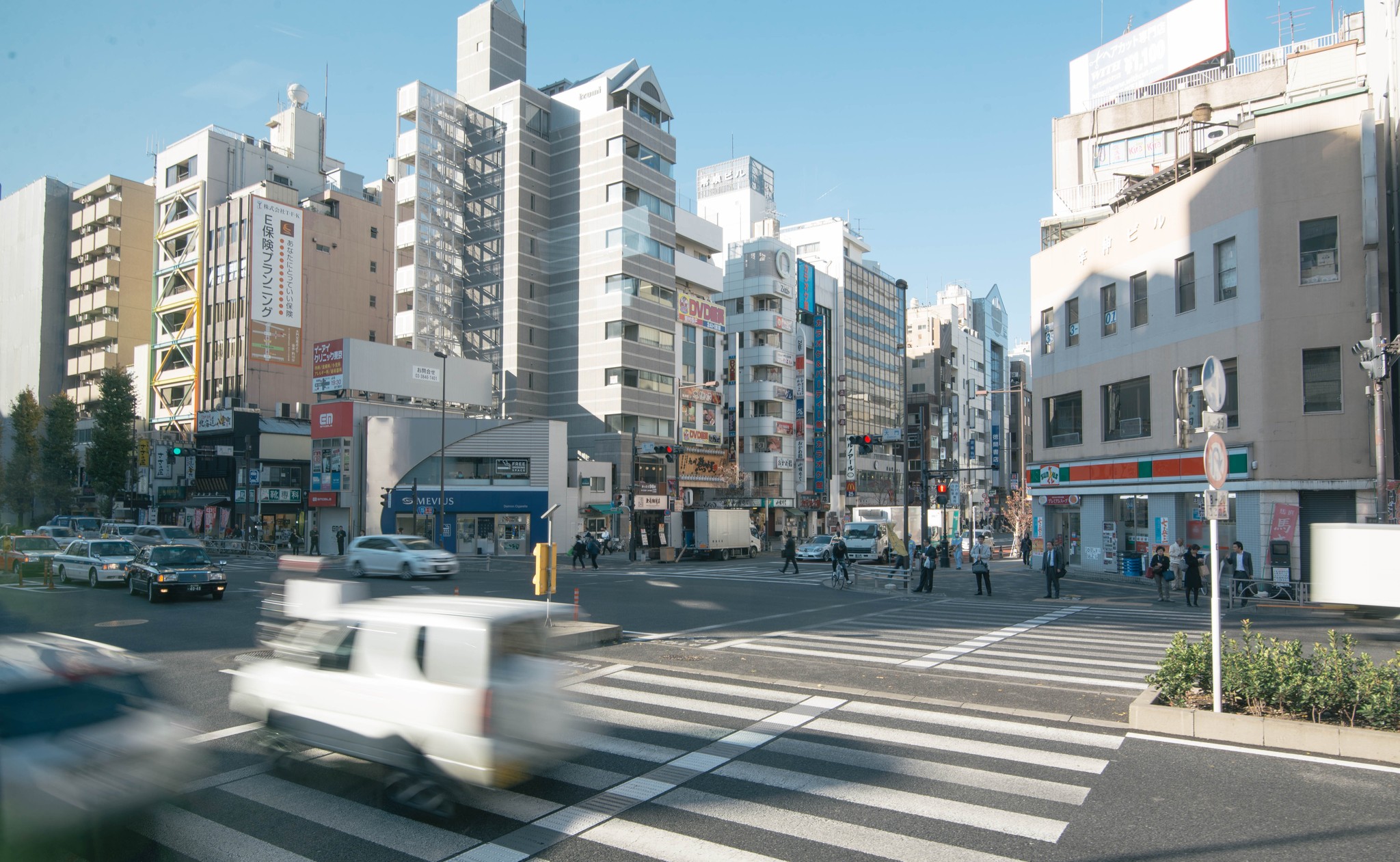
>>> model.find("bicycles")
[267,538,278,557]
[204,532,257,555]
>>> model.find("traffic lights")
[124,493,151,502]
[935,482,949,505]
[850,433,875,454]
[654,445,674,462]
[169,447,199,458]
[1350,336,1375,362]
[614,494,622,508]
[381,491,391,507]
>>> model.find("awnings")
[589,504,624,514]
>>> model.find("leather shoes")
[974,592,982,595]
[988,593,991,596]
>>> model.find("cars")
[53,534,143,590]
[794,533,843,563]
[125,542,229,605]
[1,535,62,579]
[0,615,209,854]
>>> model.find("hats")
[839,540,845,549]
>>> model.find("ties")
[1047,552,1051,568]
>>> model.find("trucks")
[842,506,926,562]
[669,505,760,562]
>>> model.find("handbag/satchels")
[1056,565,1067,578]
[1152,566,1162,574]
[1198,561,1209,576]
[972,557,988,575]
[286,542,292,548]
[1029,547,1031,550]
[1145,564,1154,579]
[566,548,579,557]
[1246,576,1258,597]
[922,555,935,570]
[297,537,305,544]
[1019,552,1022,557]
[781,549,786,558]
[1163,567,1175,581]
[1178,558,1189,571]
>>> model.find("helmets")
[954,530,959,534]
[908,533,912,537]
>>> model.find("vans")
[249,583,584,813]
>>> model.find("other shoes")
[1055,595,1059,598]
[1240,603,1245,608]
[1024,562,1029,565]
[293,552,299,555]
[581,568,585,572]
[319,553,321,556]
[955,567,961,570]
[1226,604,1234,608]
[309,554,313,556]
[926,590,930,593]
[1044,595,1051,598]
[610,551,612,555]
[336,553,343,556]
[913,588,922,592]
[601,553,605,556]
[569,567,576,571]
[590,567,599,570]
[778,568,784,574]
[793,571,799,574]
[1172,587,1187,590]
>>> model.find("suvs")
[53,514,105,540]
[100,518,140,543]
[34,526,82,551]
[958,528,995,555]
[134,523,206,555]
[347,535,459,582]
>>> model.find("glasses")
[923,541,928,542]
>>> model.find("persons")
[979,517,1013,530]
[951,530,963,570]
[154,550,197,566]
[1042,541,1062,599]
[1020,533,1032,566]
[836,522,842,532]
[571,527,613,571]
[911,537,937,593]
[779,534,799,574]
[831,540,853,584]
[887,539,914,581]
[455,471,529,479]
[970,535,992,596]
[751,520,766,540]
[287,525,404,556]
[900,533,916,577]
[225,524,245,544]
[1150,467,1254,609]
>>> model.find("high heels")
[1157,598,1174,602]
[1186,601,1199,608]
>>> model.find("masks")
[955,533,959,537]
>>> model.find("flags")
[887,523,909,557]
[139,505,232,543]
[1265,503,1300,567]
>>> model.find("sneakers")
[847,580,852,585]
[831,572,837,577]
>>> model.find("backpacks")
[606,531,611,541]
